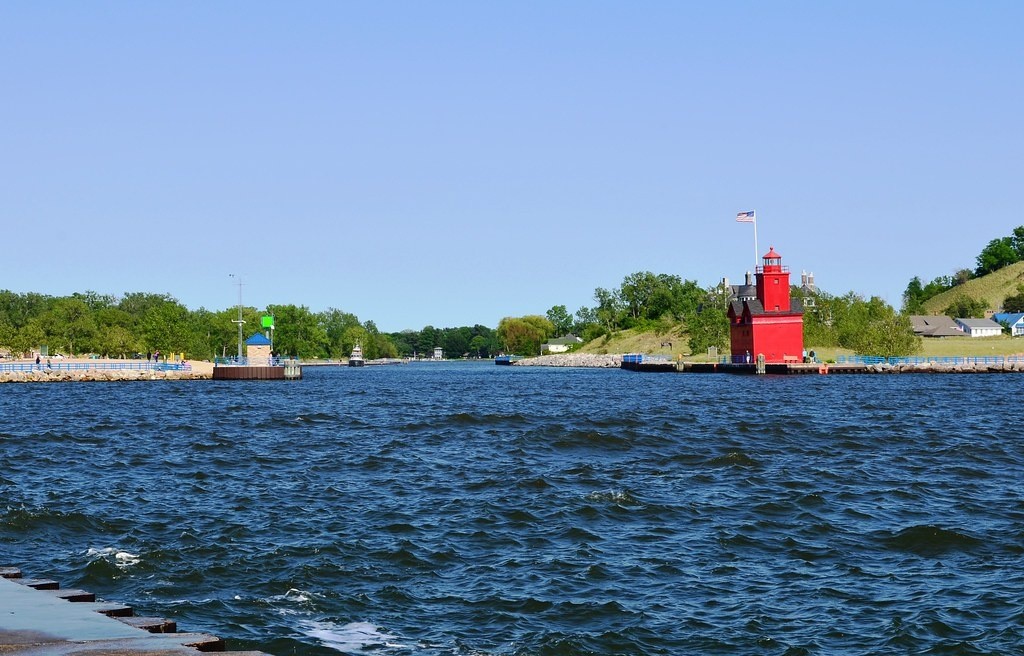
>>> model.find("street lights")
[228,274,242,363]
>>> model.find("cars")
[52,354,66,359]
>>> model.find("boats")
[495,356,509,365]
[349,345,364,366]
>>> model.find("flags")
[736,211,754,222]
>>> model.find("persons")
[36,356,41,370]
[181,361,188,366]
[269,352,280,366]
[153,352,160,362]
[147,351,151,362]
[174,360,179,369]
[802,349,807,363]
[809,349,815,364]
[745,350,751,364]
[47,359,52,369]
